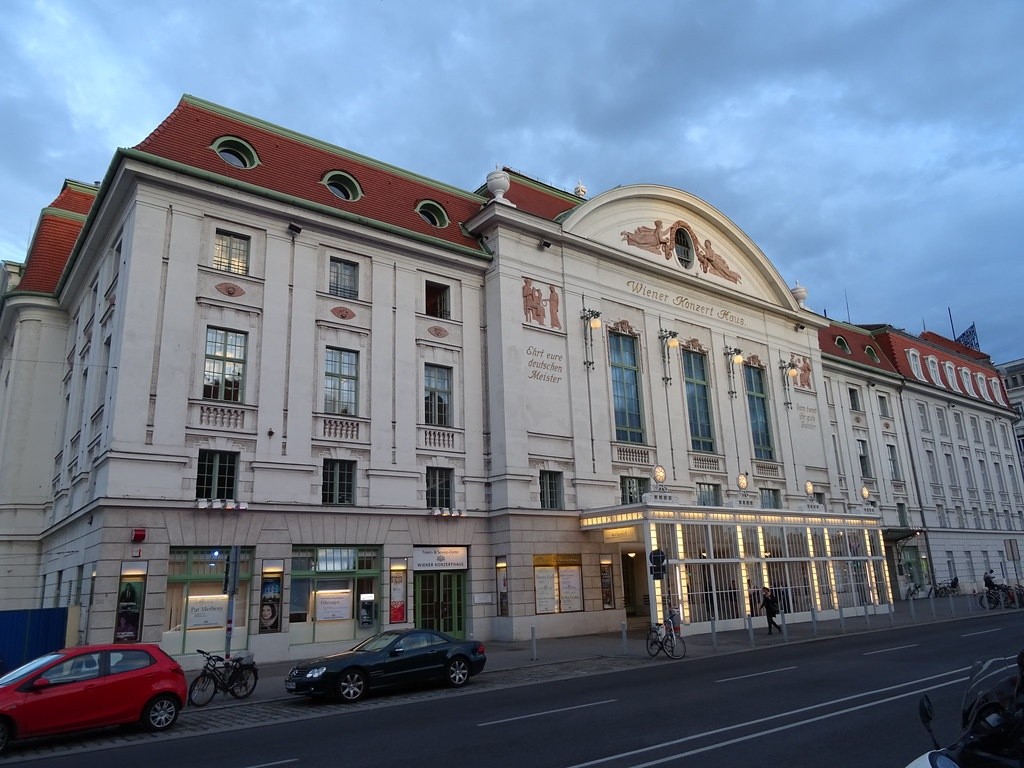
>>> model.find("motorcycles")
[903,695,1024,768]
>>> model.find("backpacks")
[766,603,780,614]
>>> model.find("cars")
[0,643,188,753]
[285,627,487,704]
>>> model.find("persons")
[120,583,136,609]
[621,220,670,247]
[759,586,782,636]
[984,570,996,590]
[747,579,761,617]
[790,354,800,386]
[797,357,813,388]
[699,238,743,284]
[544,286,562,330]
[770,582,798,613]
[261,604,278,629]
[728,580,740,619]
[522,278,545,325]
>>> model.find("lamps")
[429,507,468,517]
[195,498,247,510]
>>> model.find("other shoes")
[768,631,772,634]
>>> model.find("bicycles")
[979,583,1024,610]
[927,578,960,598]
[188,649,259,707]
[646,613,686,660]
[905,584,921,600]
